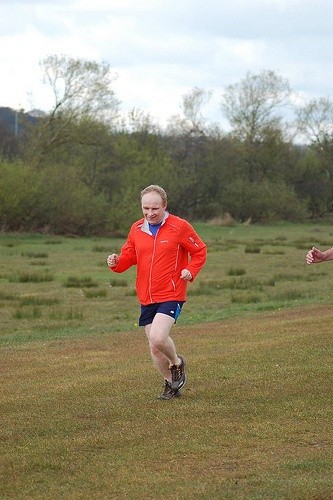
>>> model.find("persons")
[306,247,333,265]
[107,185,207,400]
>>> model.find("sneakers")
[160,379,179,400]
[168,355,186,391]
